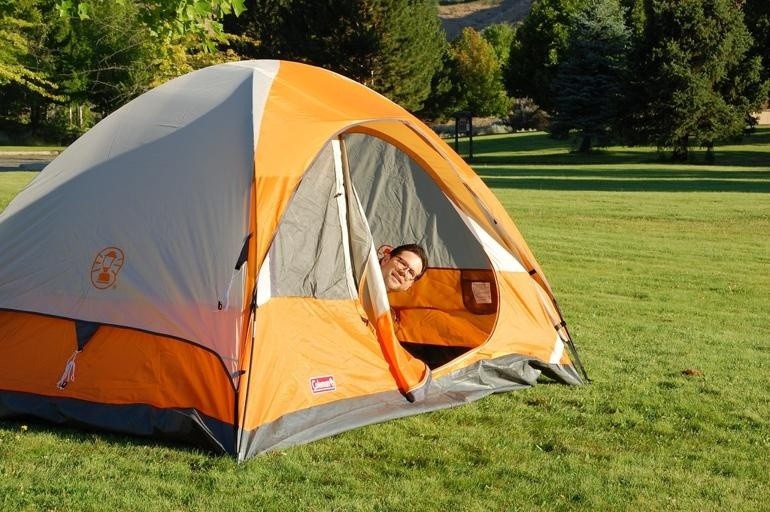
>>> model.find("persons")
[374,244,430,295]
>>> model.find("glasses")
[395,255,416,279]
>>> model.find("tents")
[0,58,593,468]
